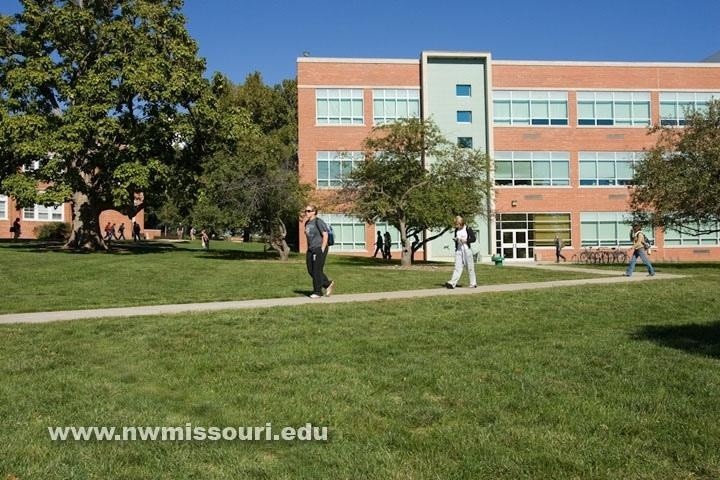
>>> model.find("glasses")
[305,210,314,213]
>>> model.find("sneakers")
[327,280,335,297]
[310,294,321,298]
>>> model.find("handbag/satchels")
[471,238,483,256]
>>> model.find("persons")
[177,222,209,251]
[554,235,566,263]
[445,215,478,288]
[304,204,334,298]
[13,218,22,243]
[104,222,143,243]
[372,231,392,260]
[622,225,656,277]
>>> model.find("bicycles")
[569,245,633,264]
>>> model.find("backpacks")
[315,217,334,245]
[635,230,651,249]
